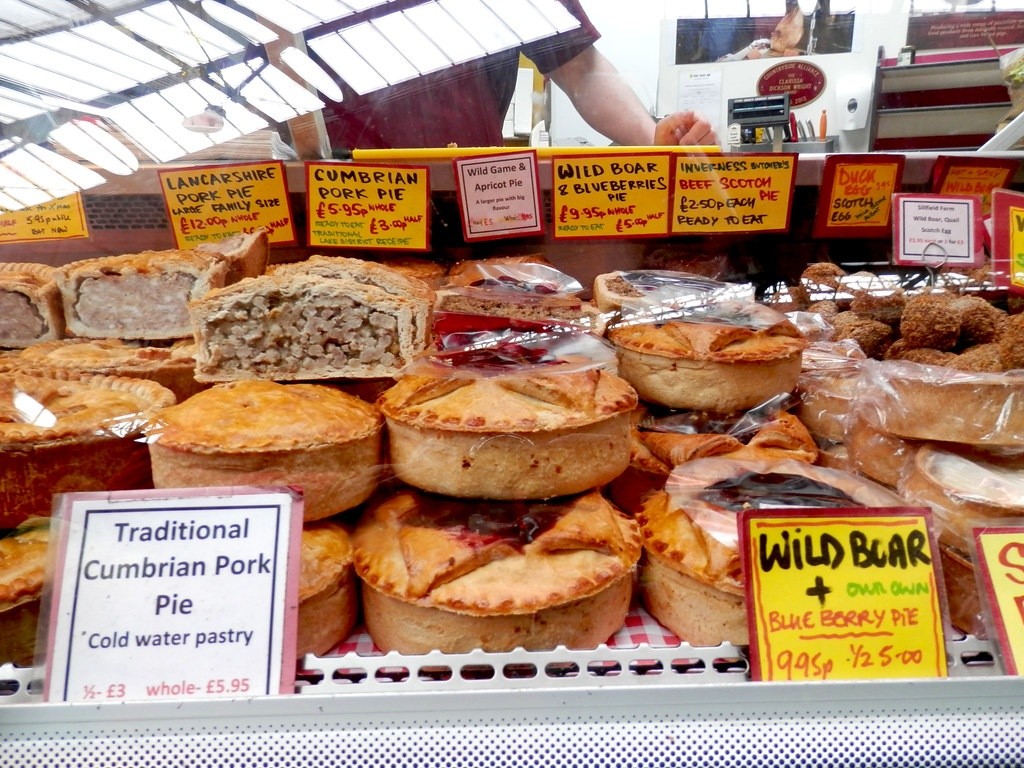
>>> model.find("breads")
[0,252,1024,655]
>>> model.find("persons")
[271,0,721,150]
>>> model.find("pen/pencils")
[805,118,815,141]
[796,120,807,141]
[789,112,798,142]
[819,110,828,141]
[783,124,791,142]
